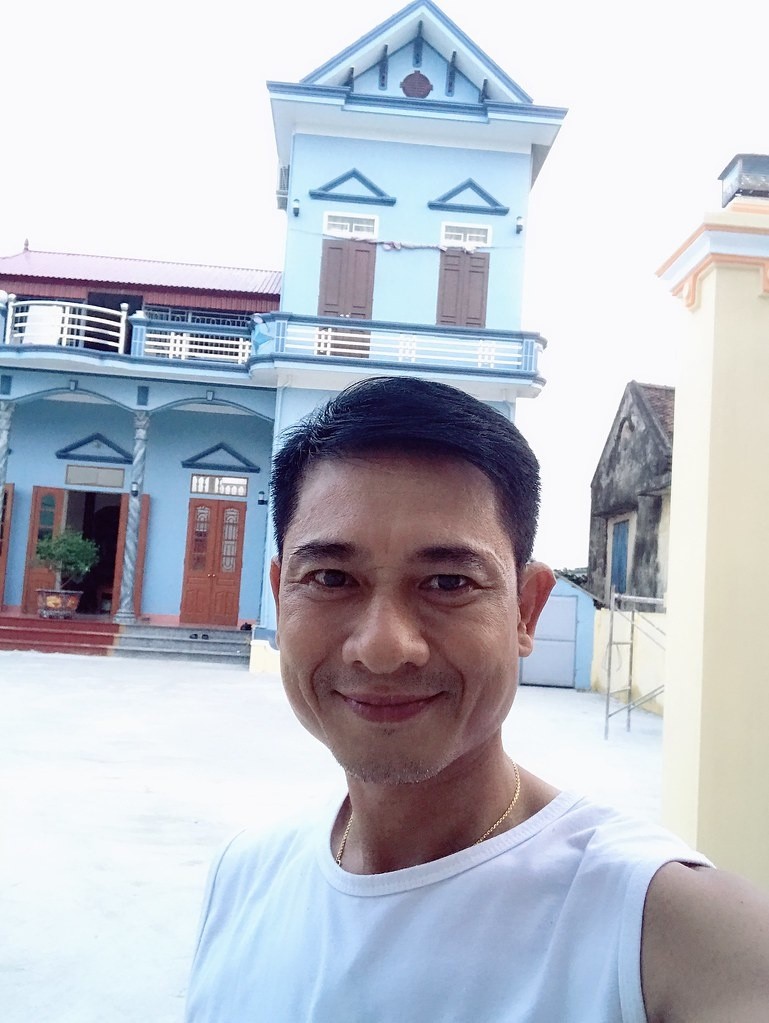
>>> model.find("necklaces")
[334,755,521,881]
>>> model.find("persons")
[184,376,769,1023]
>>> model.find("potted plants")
[31,526,100,621]
